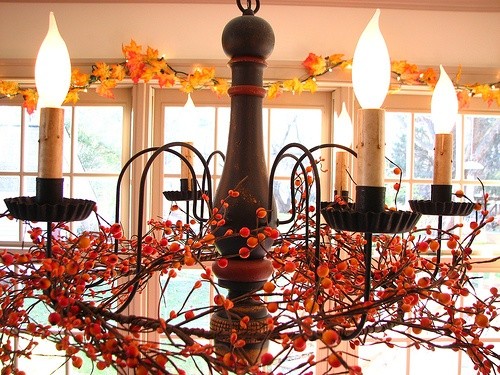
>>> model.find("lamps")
[2,0,474,367]
